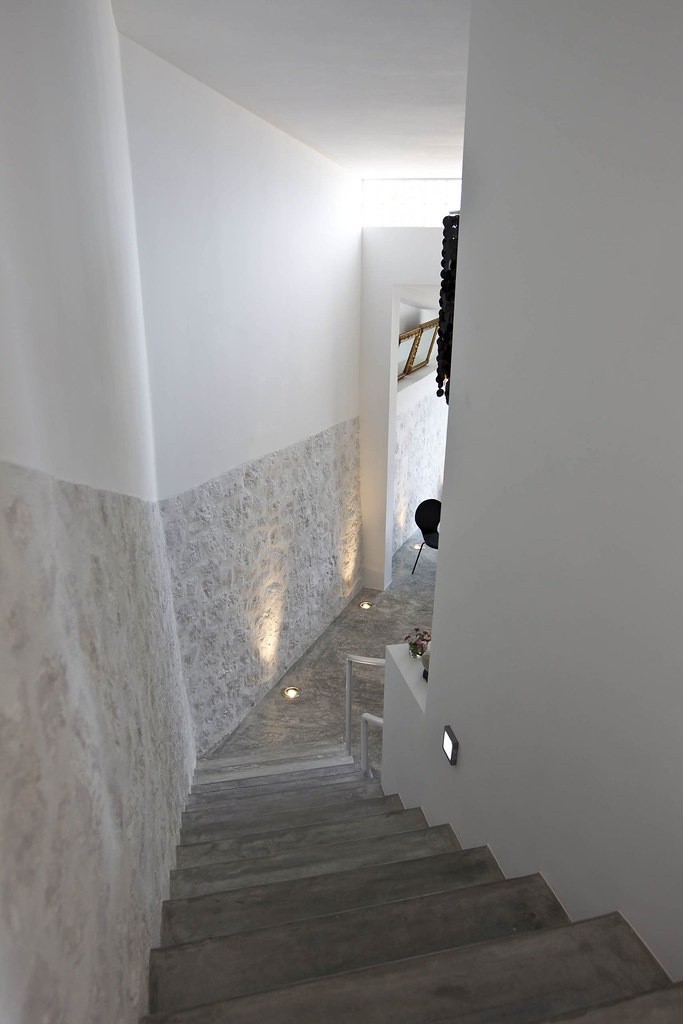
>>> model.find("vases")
[409,650,423,662]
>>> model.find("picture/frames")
[397,327,422,380]
[409,318,439,374]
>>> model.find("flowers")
[404,627,431,654]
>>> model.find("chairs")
[412,499,441,575]
